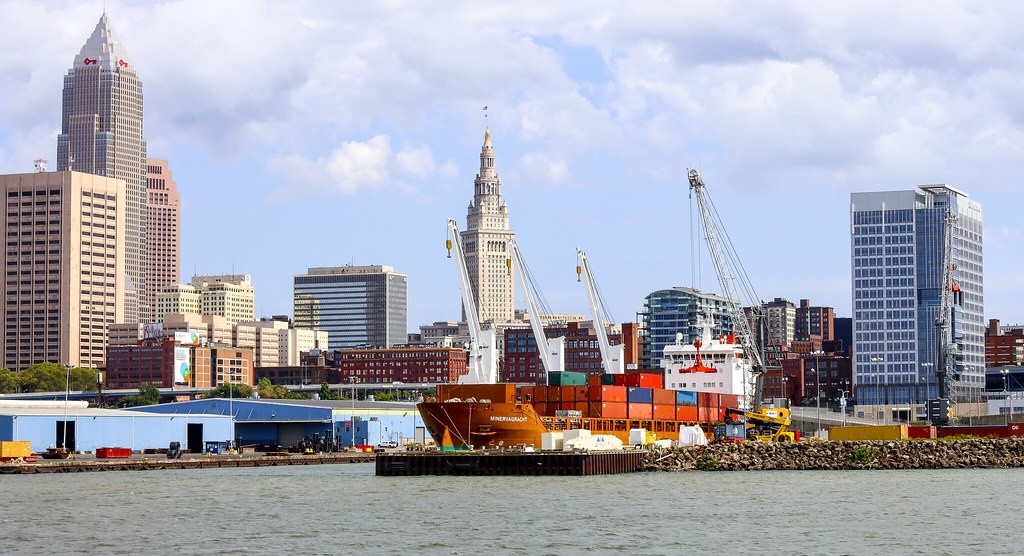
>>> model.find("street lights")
[837,389,849,427]
[225,371,236,448]
[870,357,884,427]
[964,367,975,426]
[1000,370,1010,425]
[921,363,933,426]
[810,350,824,438]
[349,376,358,447]
[61,363,76,452]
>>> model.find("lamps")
[269,415,277,421]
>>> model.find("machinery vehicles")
[444,217,499,385]
[505,239,566,387]
[574,245,627,374]
[684,167,797,444]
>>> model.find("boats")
[415,301,757,452]
